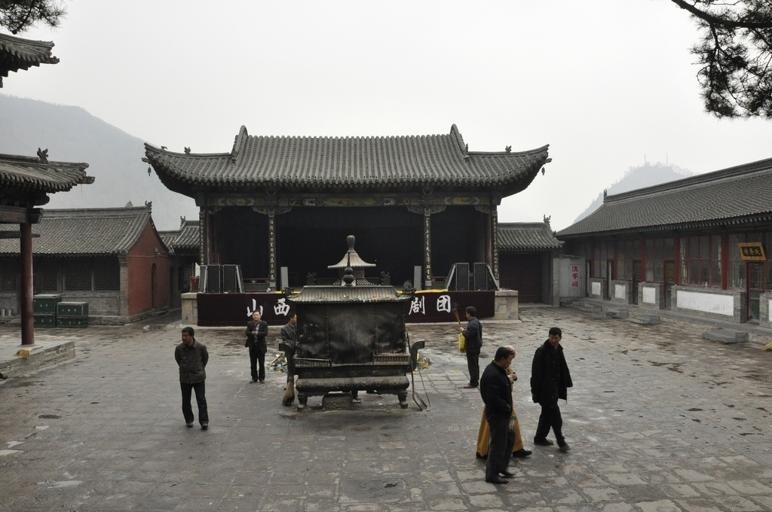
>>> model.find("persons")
[530,326,572,453]
[174,326,210,429]
[280,313,297,341]
[480,347,516,483]
[459,306,483,388]
[476,345,533,459]
[245,309,267,384]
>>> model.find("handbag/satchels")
[245,338,252,347]
[458,331,466,353]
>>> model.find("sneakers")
[186,421,193,427]
[201,423,208,429]
[476,438,571,460]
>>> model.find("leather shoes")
[501,471,515,478]
[464,382,478,388]
[490,476,510,484]
